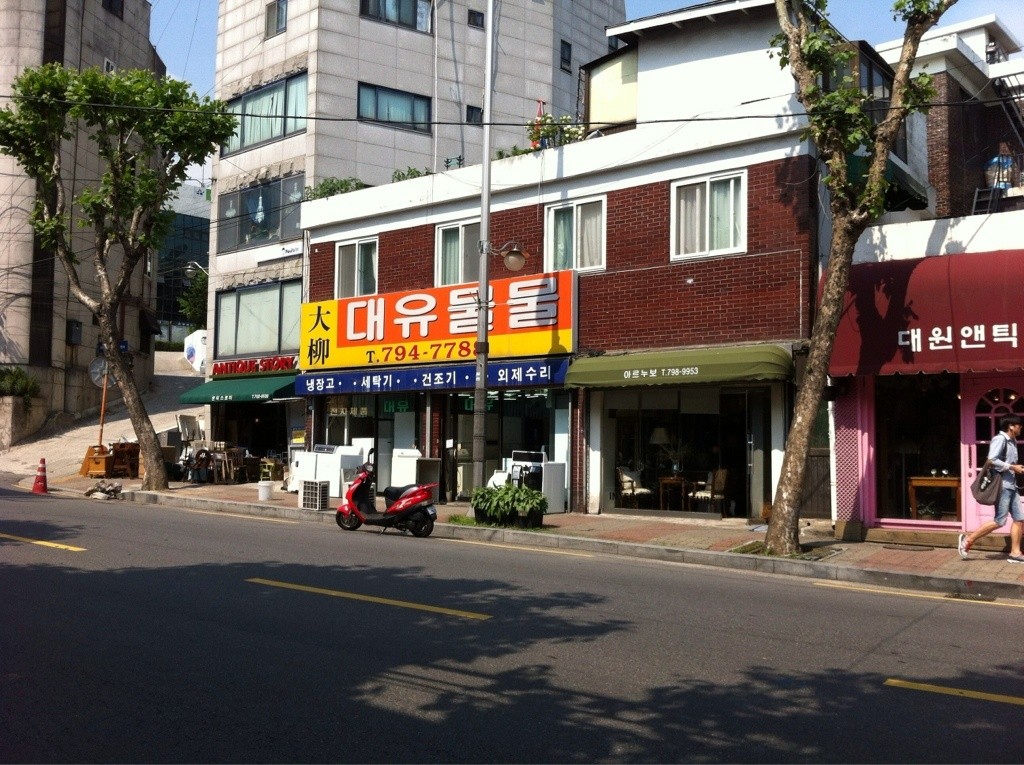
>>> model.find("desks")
[908,476,961,522]
[209,450,234,484]
[658,477,687,509]
[259,464,284,481]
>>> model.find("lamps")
[649,428,670,445]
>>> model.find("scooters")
[336,448,439,538]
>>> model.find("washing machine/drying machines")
[511,449,565,514]
[289,444,338,494]
[389,447,442,504]
[316,445,364,498]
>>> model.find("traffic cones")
[31,458,51,495]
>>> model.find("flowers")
[526,114,584,141]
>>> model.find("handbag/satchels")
[970,460,1002,505]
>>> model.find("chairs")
[615,463,654,509]
[688,469,727,515]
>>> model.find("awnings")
[819,250,1024,374]
[179,377,296,401]
[563,344,795,389]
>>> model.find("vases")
[542,137,555,148]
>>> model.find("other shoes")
[958,534,969,559]
[1007,553,1024,563]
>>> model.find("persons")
[957,413,1024,565]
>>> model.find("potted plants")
[471,485,548,527]
[661,438,691,474]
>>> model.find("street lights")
[468,240,531,517]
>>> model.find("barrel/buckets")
[257,481,275,501]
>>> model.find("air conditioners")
[343,482,377,510]
[298,480,330,511]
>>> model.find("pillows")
[623,471,642,489]
